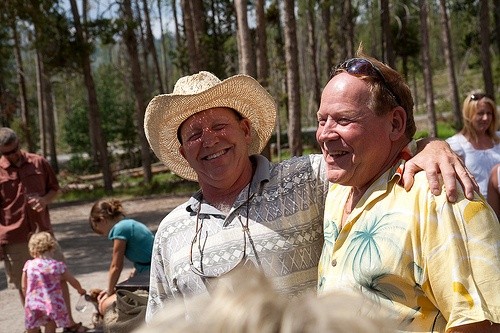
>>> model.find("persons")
[315,40,499,333]
[89,198,155,316]
[143,72,480,333]
[0,126,86,333]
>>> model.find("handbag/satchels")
[103,289,148,333]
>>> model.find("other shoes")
[63,322,90,333]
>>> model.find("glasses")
[0,141,19,155]
[329,57,401,106]
[190,216,246,278]
[469,94,494,103]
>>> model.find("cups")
[75,295,88,312]
[27,193,38,210]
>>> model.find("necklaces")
[344,185,365,214]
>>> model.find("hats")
[144,70,278,181]
